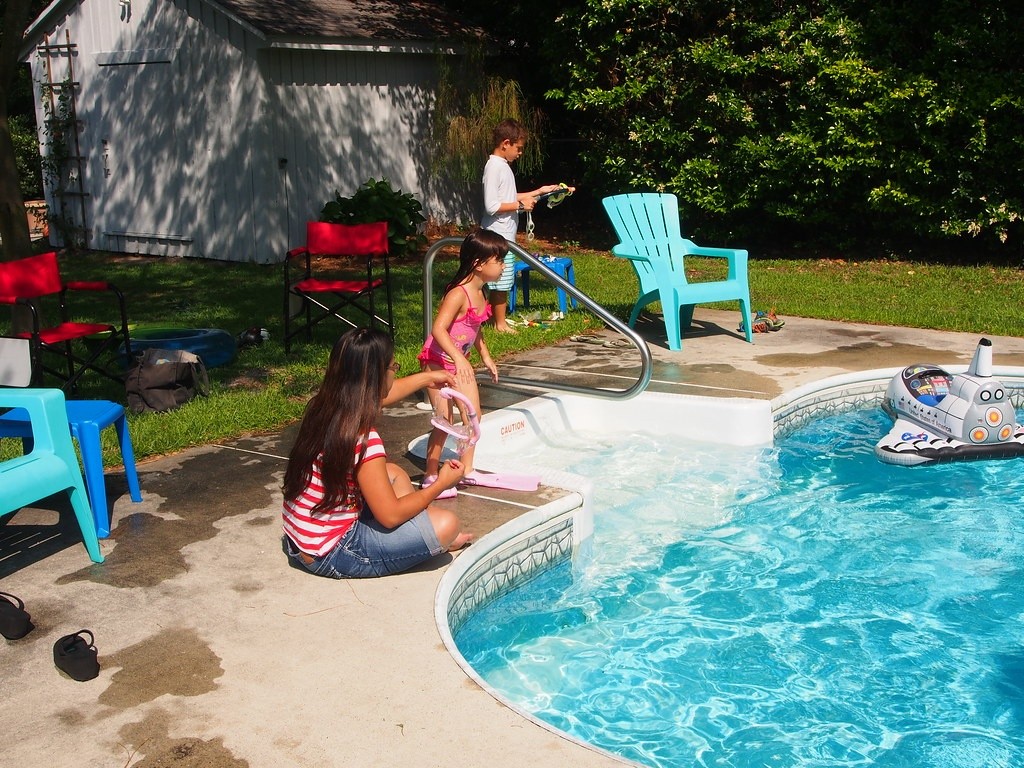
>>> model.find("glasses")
[386,362,400,372]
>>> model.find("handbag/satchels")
[125,348,209,415]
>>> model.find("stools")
[0,400,144,538]
[509,257,577,313]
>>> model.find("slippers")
[0,592,35,640]
[54,630,101,682]
[603,339,637,349]
[570,335,604,344]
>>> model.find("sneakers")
[739,317,768,334]
[755,307,785,330]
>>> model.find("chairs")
[1,389,104,563]
[0,252,133,399]
[285,221,394,355]
[602,193,753,351]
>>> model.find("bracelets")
[519,200,524,211]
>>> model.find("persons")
[481,118,562,334]
[418,229,540,499]
[279,326,475,579]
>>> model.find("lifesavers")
[117,326,236,370]
